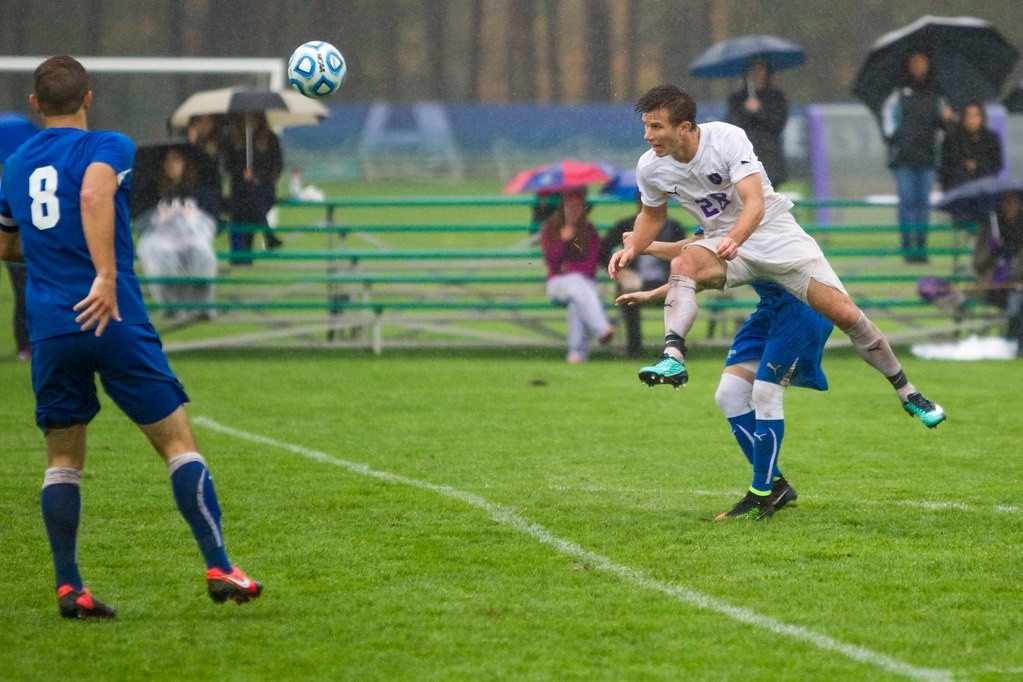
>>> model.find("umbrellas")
[686,35,809,97]
[933,171,1023,243]
[601,165,682,206]
[850,17,1023,121]
[171,82,330,171]
[129,127,223,211]
[504,161,622,227]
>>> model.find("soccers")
[287,40,349,101]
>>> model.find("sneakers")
[205,567,261,601]
[639,351,689,390]
[768,478,797,510]
[715,489,775,522]
[901,392,946,429]
[57,586,115,619]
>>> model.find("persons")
[615,217,838,525]
[936,101,1006,193]
[607,86,946,428]
[724,58,792,192]
[539,186,616,365]
[600,189,687,363]
[185,112,285,270]
[0,56,261,618]
[974,193,1023,357]
[880,49,960,264]
[0,114,40,365]
[137,150,221,319]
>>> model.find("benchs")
[133,197,1001,355]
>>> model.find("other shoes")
[598,328,614,345]
[572,353,583,362]
